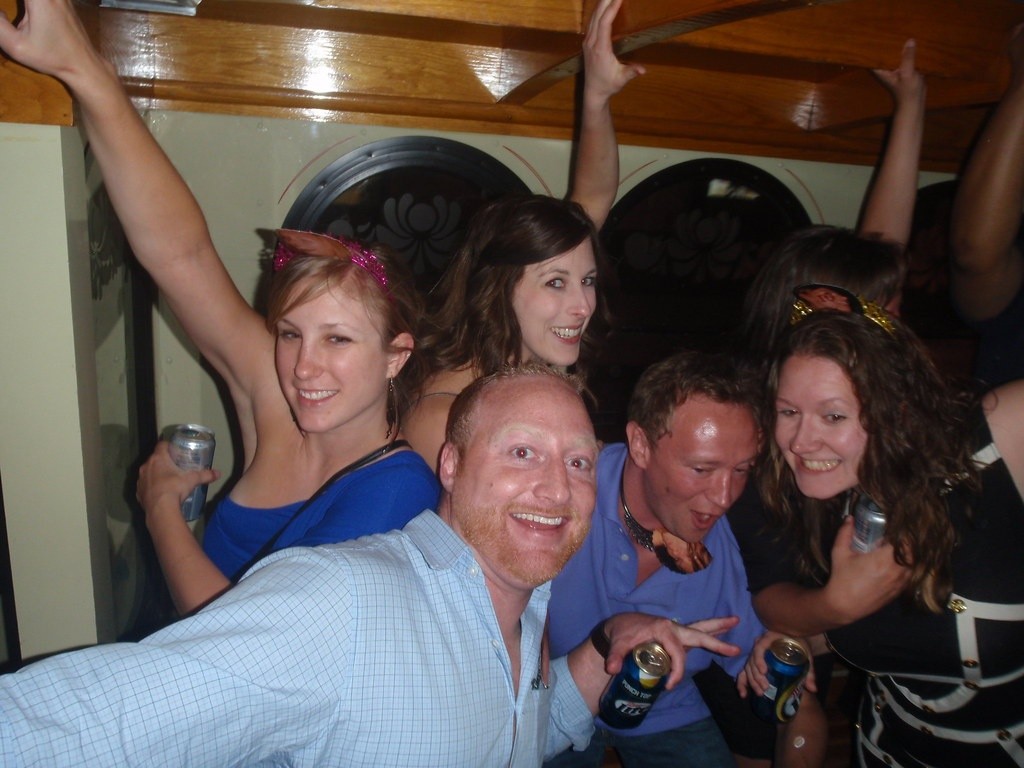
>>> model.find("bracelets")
[590,618,612,658]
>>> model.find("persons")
[0,1,1024,768]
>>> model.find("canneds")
[599,640,672,730]
[161,424,215,523]
[853,498,884,553]
[758,638,808,724]
